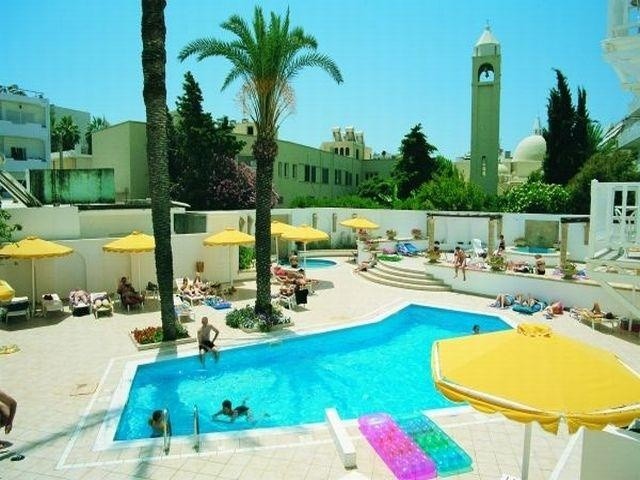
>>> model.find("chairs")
[568,306,617,333]
[1,262,315,325]
[384,239,493,260]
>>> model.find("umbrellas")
[269,220,298,264]
[339,216,381,231]
[202,226,255,286]
[429,322,639,479]
[101,230,156,295]
[281,224,329,266]
[0,236,74,318]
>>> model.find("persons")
[585,298,615,320]
[147,410,169,438]
[0,389,16,435]
[269,250,312,299]
[197,316,219,368]
[70,278,145,315]
[210,399,268,423]
[147,273,226,305]
[478,235,546,275]
[452,246,467,281]
[491,291,564,319]
[434,241,440,252]
